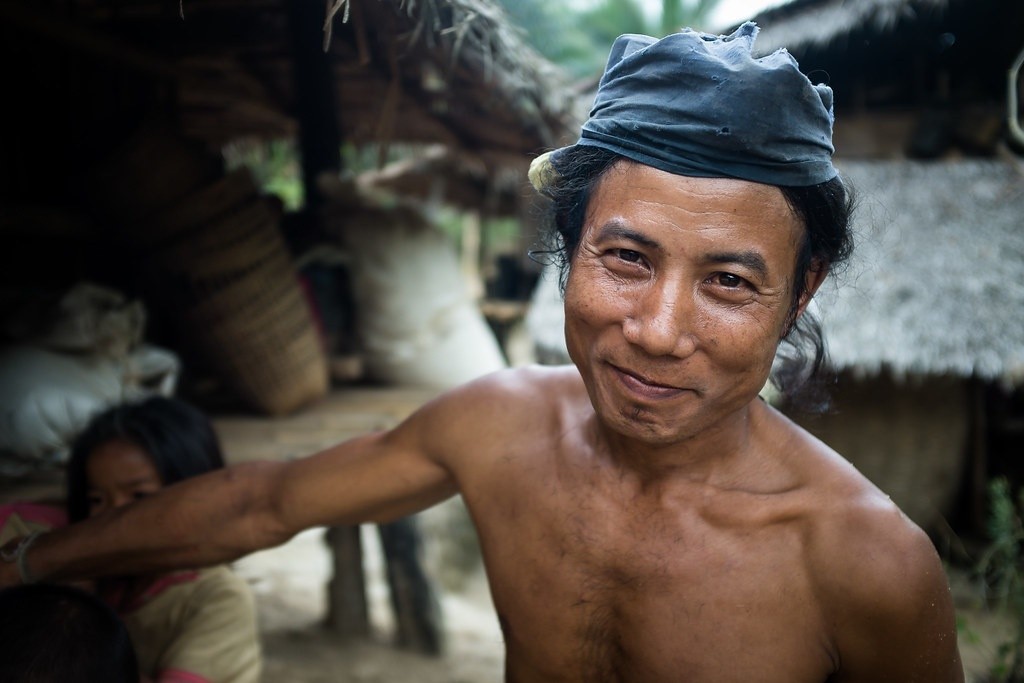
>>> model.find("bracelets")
[1,529,40,588]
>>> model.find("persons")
[0,392,262,683]
[0,22,965,683]
